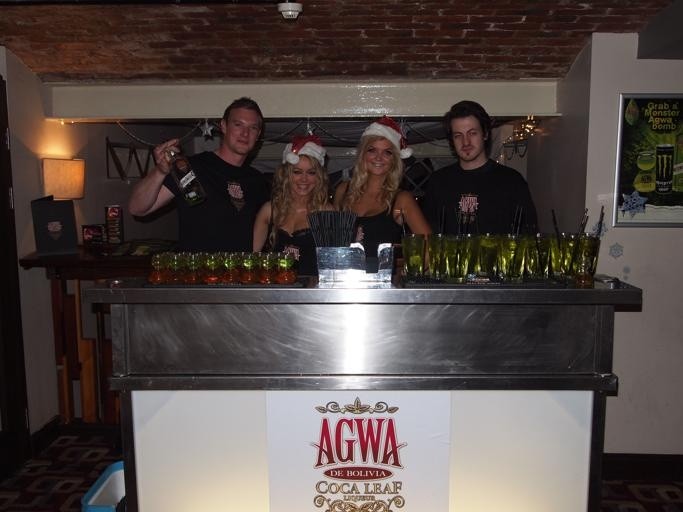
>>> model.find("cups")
[400,232,600,283]
[145,251,297,286]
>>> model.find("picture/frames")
[611,92,683,229]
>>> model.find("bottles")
[672,133,683,192]
[165,147,206,206]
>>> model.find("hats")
[281,134,326,167]
[361,115,413,160]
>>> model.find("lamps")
[40,158,84,199]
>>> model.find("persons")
[129,97,272,252]
[333,114,434,256]
[417,100,541,235]
[252,135,340,275]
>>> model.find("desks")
[19,239,186,424]
